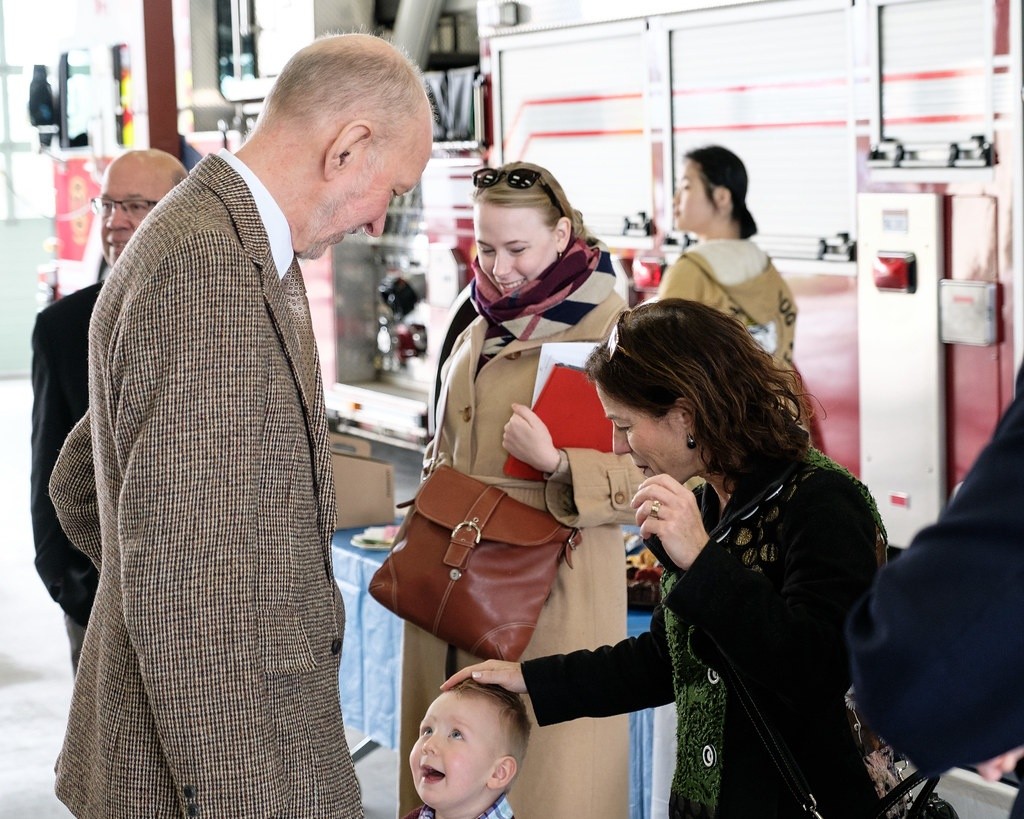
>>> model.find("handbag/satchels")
[368,464,584,662]
[869,770,958,819]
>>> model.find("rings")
[651,497,660,517]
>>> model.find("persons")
[653,147,800,381]
[396,160,709,818]
[54,31,432,819]
[27,144,191,667]
[849,358,1024,819]
[406,680,531,819]
[27,63,60,145]
[439,298,889,818]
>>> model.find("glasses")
[605,296,662,365]
[90,197,158,218]
[472,168,565,217]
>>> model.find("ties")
[281,254,318,418]
